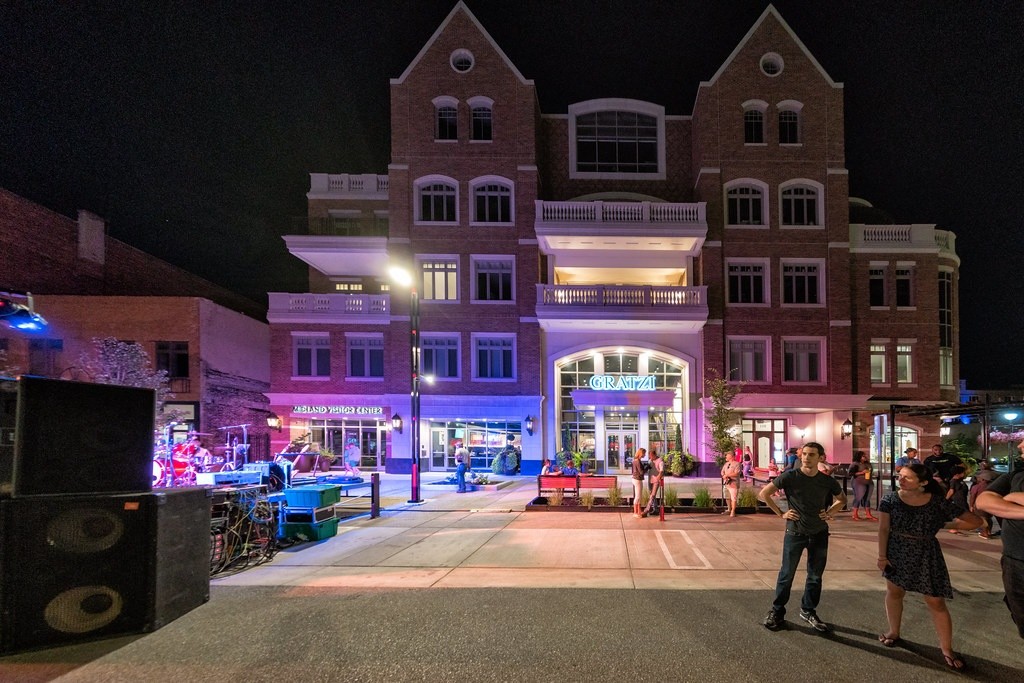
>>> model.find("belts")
[785,528,828,537]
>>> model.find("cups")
[865,470,870,479]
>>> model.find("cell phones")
[884,565,895,575]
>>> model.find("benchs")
[753,476,769,486]
[537,474,617,497]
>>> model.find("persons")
[349,443,361,477]
[757,443,846,633]
[642,451,665,517]
[733,443,742,464]
[548,460,593,475]
[632,448,648,518]
[922,444,1002,540]
[846,451,878,521]
[344,445,353,477]
[974,441,1024,638]
[194,439,209,456]
[720,450,741,516]
[541,459,552,475]
[896,448,921,473]
[742,446,757,481]
[780,449,798,500]
[793,448,804,470]
[454,442,471,493]
[817,452,836,519]
[877,462,964,671]
[769,457,779,496]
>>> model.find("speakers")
[11,376,157,492]
[2,484,215,648]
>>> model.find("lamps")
[392,412,402,434]
[266,412,282,432]
[579,452,590,473]
[525,415,533,436]
[840,418,853,440]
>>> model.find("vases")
[297,456,313,473]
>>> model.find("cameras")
[725,477,731,484]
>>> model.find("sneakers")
[800,608,828,631]
[763,608,787,627]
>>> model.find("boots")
[641,495,656,517]
[650,497,659,515]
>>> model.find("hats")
[724,450,735,455]
[973,473,993,481]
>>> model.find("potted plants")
[317,447,335,472]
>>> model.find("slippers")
[940,651,963,668]
[878,633,900,645]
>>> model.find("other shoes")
[867,517,879,521]
[989,530,1002,538]
[852,514,860,521]
[721,510,731,515]
[633,513,642,518]
[978,530,991,538]
[729,514,736,518]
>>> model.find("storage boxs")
[282,484,342,541]
